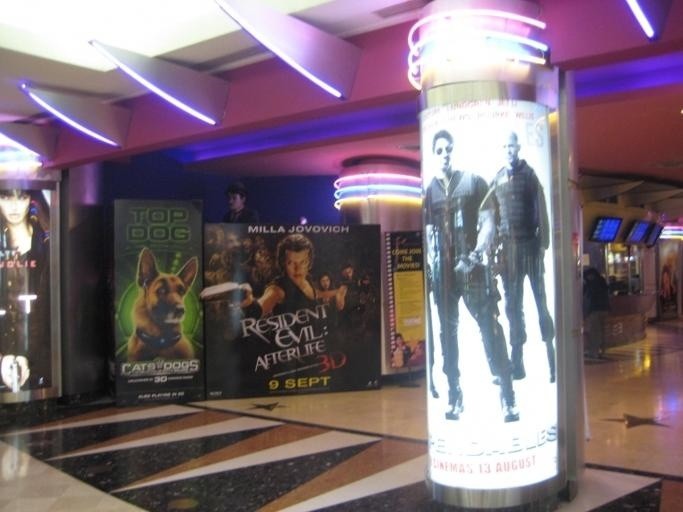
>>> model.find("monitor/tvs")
[645,223,664,248]
[623,219,652,244]
[589,216,623,243]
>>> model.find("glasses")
[435,144,453,155]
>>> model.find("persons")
[425,263,440,400]
[488,131,556,387]
[0,188,51,387]
[392,333,410,361]
[583,266,611,357]
[659,264,671,298]
[406,340,426,366]
[424,128,520,423]
[668,250,678,295]
[206,224,379,390]
[222,182,259,224]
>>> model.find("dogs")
[125,247,200,360]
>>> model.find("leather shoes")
[499,390,520,423]
[491,359,526,385]
[444,386,464,421]
[547,349,556,383]
